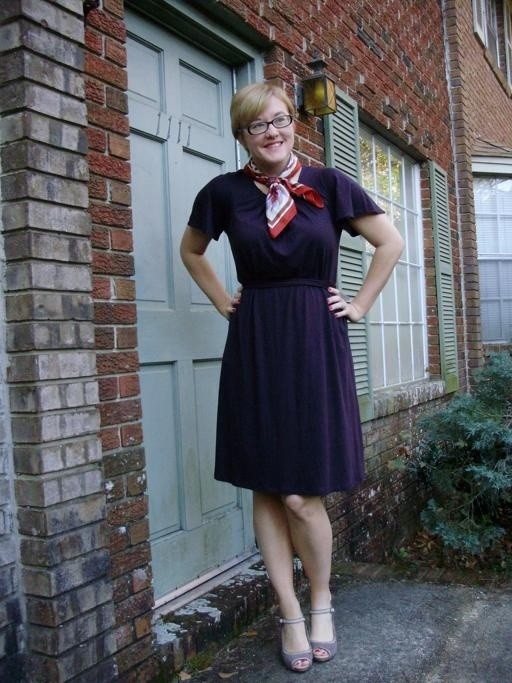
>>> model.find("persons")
[178,83,409,673]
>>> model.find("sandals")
[277,616,313,674]
[308,606,338,663]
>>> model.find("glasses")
[240,114,292,136]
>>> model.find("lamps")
[296,58,337,116]
